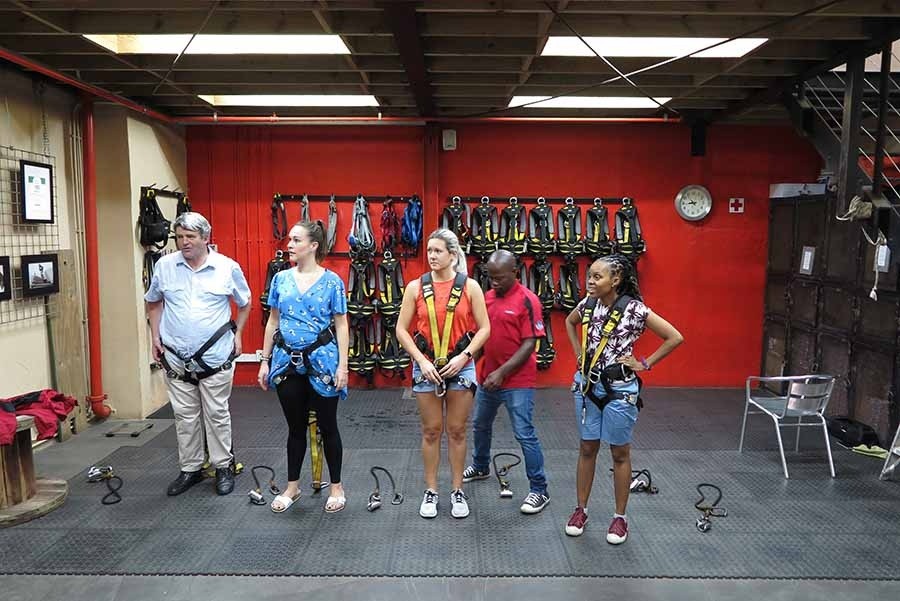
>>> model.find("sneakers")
[419,489,439,517]
[565,507,590,536]
[450,488,470,518]
[520,492,551,513]
[607,517,629,544]
[462,466,491,482]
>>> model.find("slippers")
[325,490,346,513]
[270,491,303,512]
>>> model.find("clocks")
[675,184,712,220]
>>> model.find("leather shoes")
[215,467,236,495]
[167,470,203,495]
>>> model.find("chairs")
[739,374,842,478]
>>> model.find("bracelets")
[642,358,651,370]
[575,352,583,360]
[260,353,272,360]
[462,350,472,360]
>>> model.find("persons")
[144,212,252,496]
[565,253,684,544]
[396,229,491,518]
[462,250,551,513]
[257,219,348,512]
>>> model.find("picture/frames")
[19,160,56,224]
[19,254,60,299]
[0,255,12,301]
